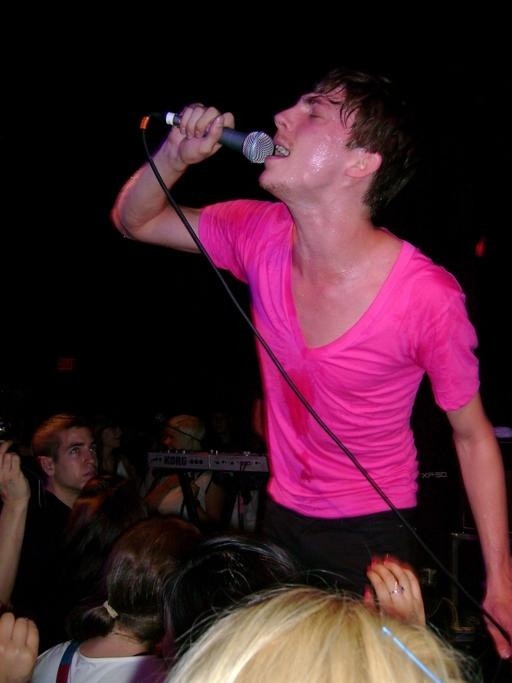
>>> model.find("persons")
[108,61,512,661]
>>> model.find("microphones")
[152,110,274,164]
